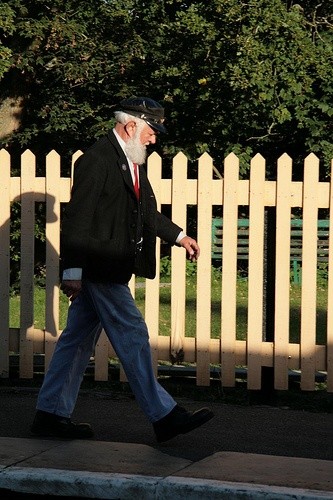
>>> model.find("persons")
[32,96,216,443]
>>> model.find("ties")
[133,163,140,203]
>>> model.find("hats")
[117,96,166,133]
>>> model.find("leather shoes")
[30,410,92,440]
[154,404,214,447]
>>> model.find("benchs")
[211,218,329,286]
